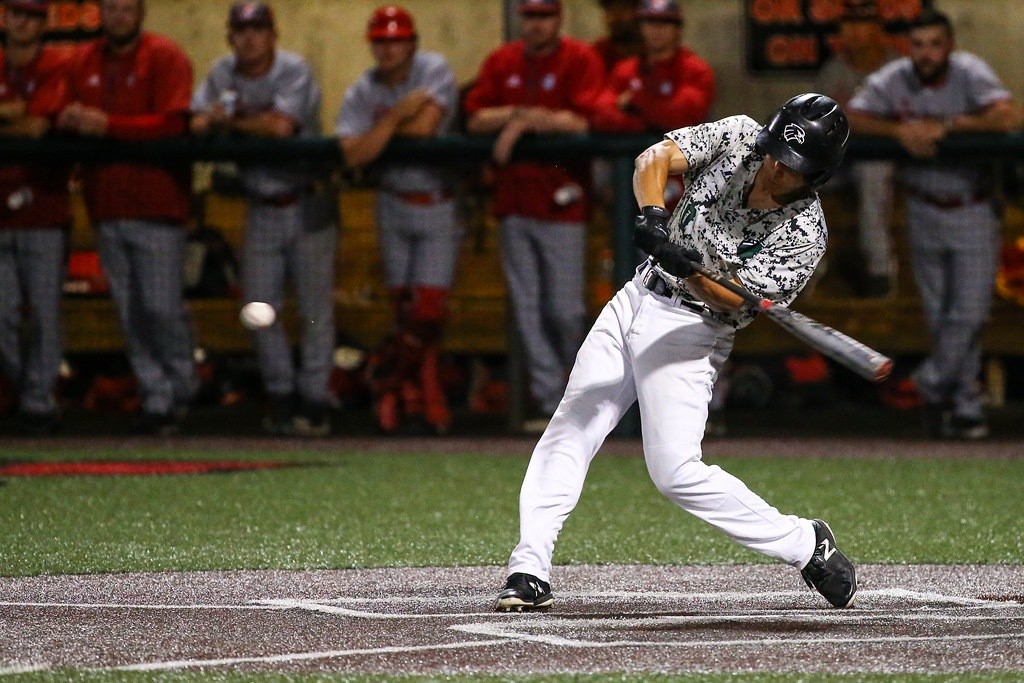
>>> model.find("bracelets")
[942,115,955,134]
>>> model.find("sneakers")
[799,518,857,609]
[493,573,555,611]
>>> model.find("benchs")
[59,134,1024,357]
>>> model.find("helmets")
[756,92,850,188]
[364,5,415,41]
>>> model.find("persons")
[814,12,904,278]
[494,90,859,613]
[592,0,718,217]
[188,0,341,439]
[462,0,606,434]
[338,5,460,437]
[46,0,194,440]
[842,11,1020,442]
[0,0,75,440]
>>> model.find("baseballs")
[240,301,276,330]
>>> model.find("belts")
[911,187,985,209]
[404,193,448,205]
[638,263,704,313]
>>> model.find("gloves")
[657,241,701,279]
[632,206,671,254]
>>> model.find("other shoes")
[265,403,328,436]
[520,410,552,432]
[944,415,988,441]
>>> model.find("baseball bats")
[630,214,896,381]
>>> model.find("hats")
[228,1,272,28]
[637,0,682,22]
[519,0,561,16]
